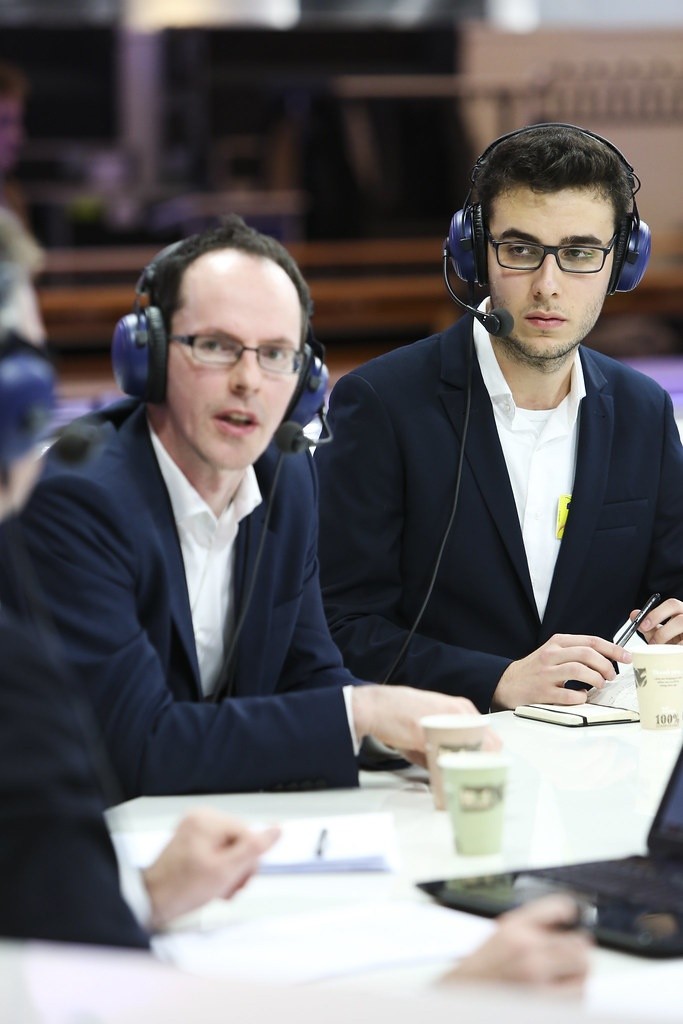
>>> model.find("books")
[512,673,641,727]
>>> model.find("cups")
[630,644,683,728]
[420,712,486,811]
[437,752,507,856]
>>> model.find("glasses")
[169,334,304,374]
[481,228,617,273]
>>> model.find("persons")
[309,124,683,712]
[0,207,588,1024]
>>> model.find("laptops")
[435,742,683,960]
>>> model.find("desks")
[99,699,683,1024]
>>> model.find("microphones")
[273,408,334,453]
[443,258,515,338]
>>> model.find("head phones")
[444,123,652,296]
[111,240,329,429]
[1,240,61,466]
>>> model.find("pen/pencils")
[610,593,661,666]
[432,884,653,958]
